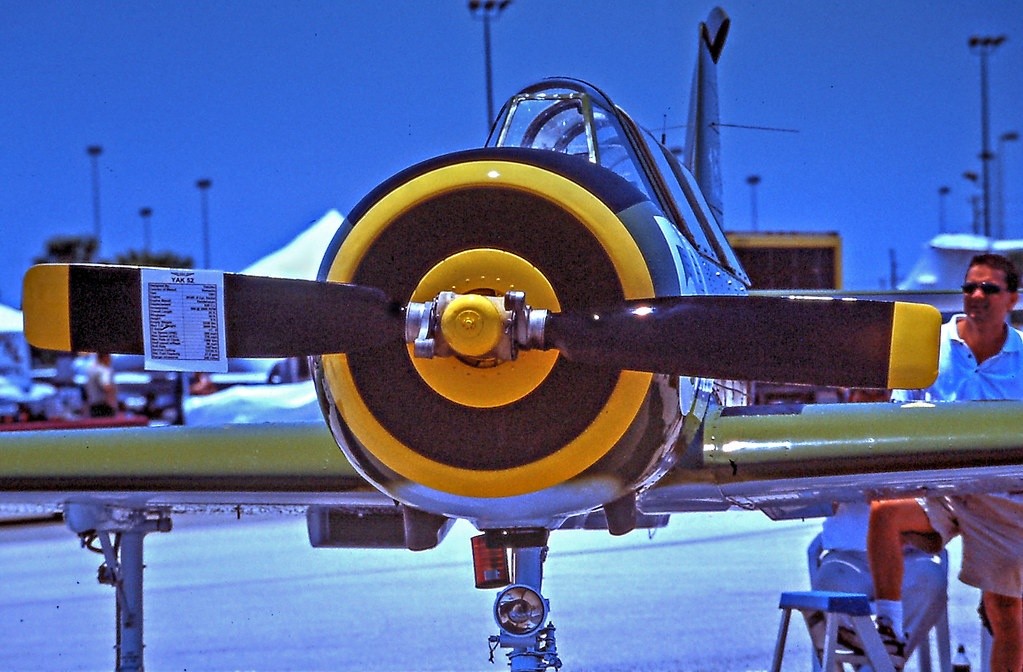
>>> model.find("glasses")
[960,282,1010,296]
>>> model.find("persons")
[191,373,215,394]
[87,352,118,418]
[836,254,1023,672]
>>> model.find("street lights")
[968,35,1007,236]
[467,0,512,135]
[197,179,211,271]
[140,207,153,253]
[996,132,1018,240]
[746,176,760,231]
[938,152,997,237]
[86,146,104,242]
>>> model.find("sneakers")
[837,615,909,671]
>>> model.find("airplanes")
[0,5,1021,670]
